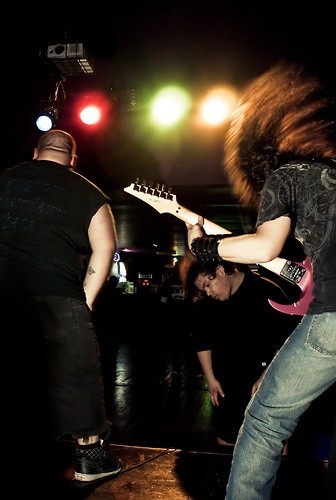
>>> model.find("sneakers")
[74,451,122,481]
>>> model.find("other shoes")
[191,434,234,452]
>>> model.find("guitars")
[123,179,313,318]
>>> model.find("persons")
[222,61,336,499]
[0,129,124,482]
[192,256,301,447]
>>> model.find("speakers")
[67,43,83,57]
[47,44,67,58]
